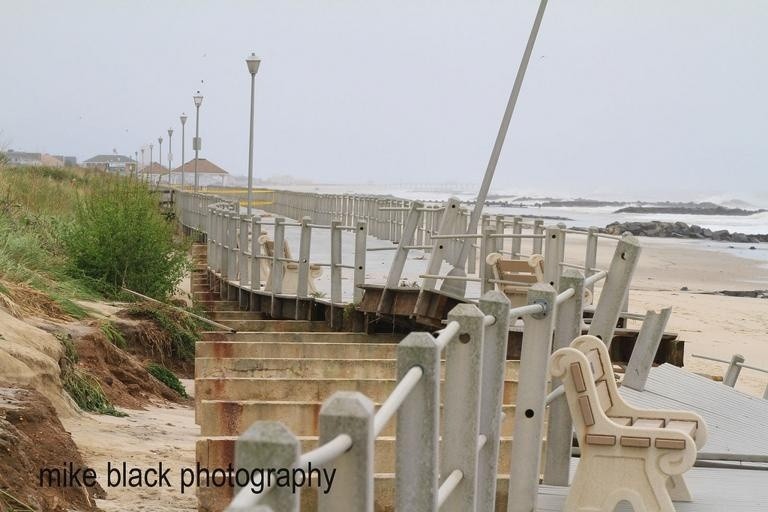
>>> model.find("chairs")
[548,334,707,512]
[485,251,546,307]
[227,226,323,297]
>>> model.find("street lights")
[192,89,204,198]
[127,127,175,193]
[179,113,187,190]
[242,52,262,226]
